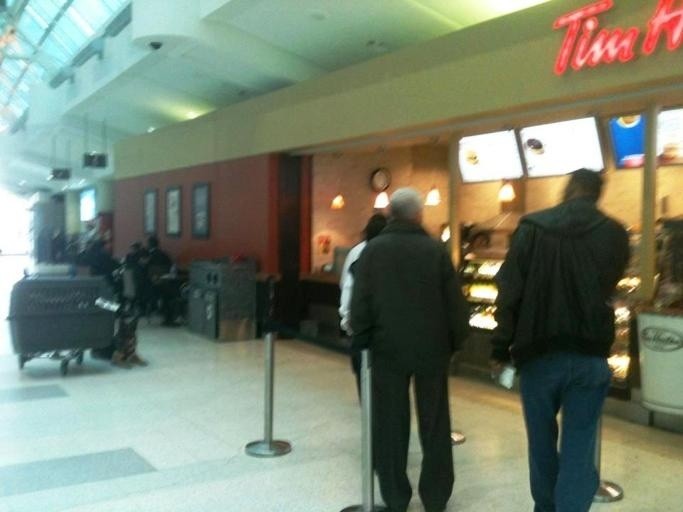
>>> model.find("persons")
[35,211,172,357]
[489,168,630,512]
[338,187,470,510]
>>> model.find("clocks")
[371,167,392,193]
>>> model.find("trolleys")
[16,264,83,378]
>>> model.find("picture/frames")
[193,183,210,239]
[144,188,159,234]
[166,186,181,236]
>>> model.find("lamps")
[425,184,442,206]
[374,190,390,210]
[330,192,345,212]
[498,179,517,203]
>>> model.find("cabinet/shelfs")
[459,248,639,403]
